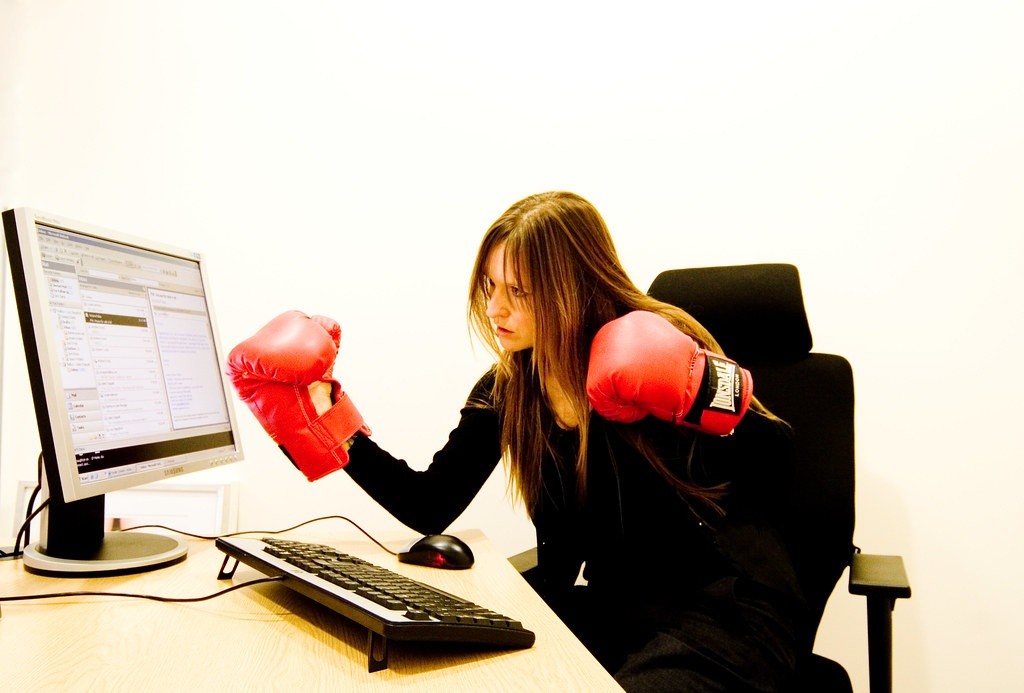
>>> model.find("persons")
[224,190,806,693]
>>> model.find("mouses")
[399,534,474,569]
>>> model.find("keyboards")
[216,539,536,671]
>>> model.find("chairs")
[507,264,911,693]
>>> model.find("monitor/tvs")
[3,207,237,576]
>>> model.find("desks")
[0,527,629,693]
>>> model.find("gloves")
[586,310,753,437]
[227,309,372,482]
